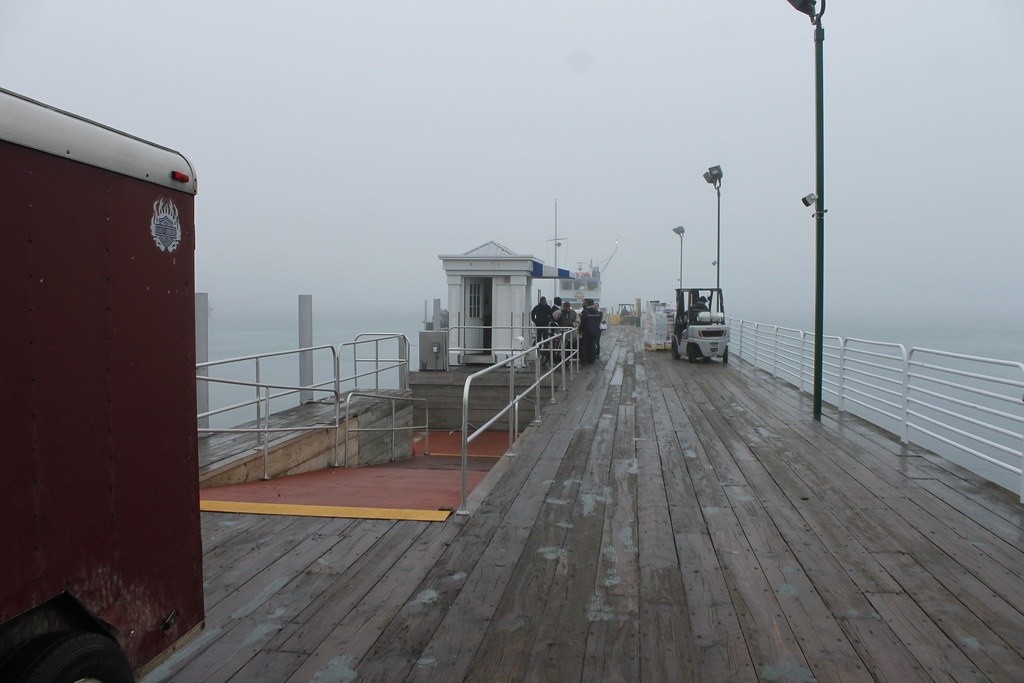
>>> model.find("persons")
[621,306,630,316]
[683,296,709,322]
[531,295,601,364]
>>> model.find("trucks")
[0,88,205,682]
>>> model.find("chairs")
[691,309,706,324]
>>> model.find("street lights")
[703,164,724,326]
[671,225,685,288]
[787,0,826,421]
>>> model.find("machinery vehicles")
[671,287,730,364]
[609,303,636,325]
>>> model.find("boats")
[424,309,450,329]
[558,260,602,317]
